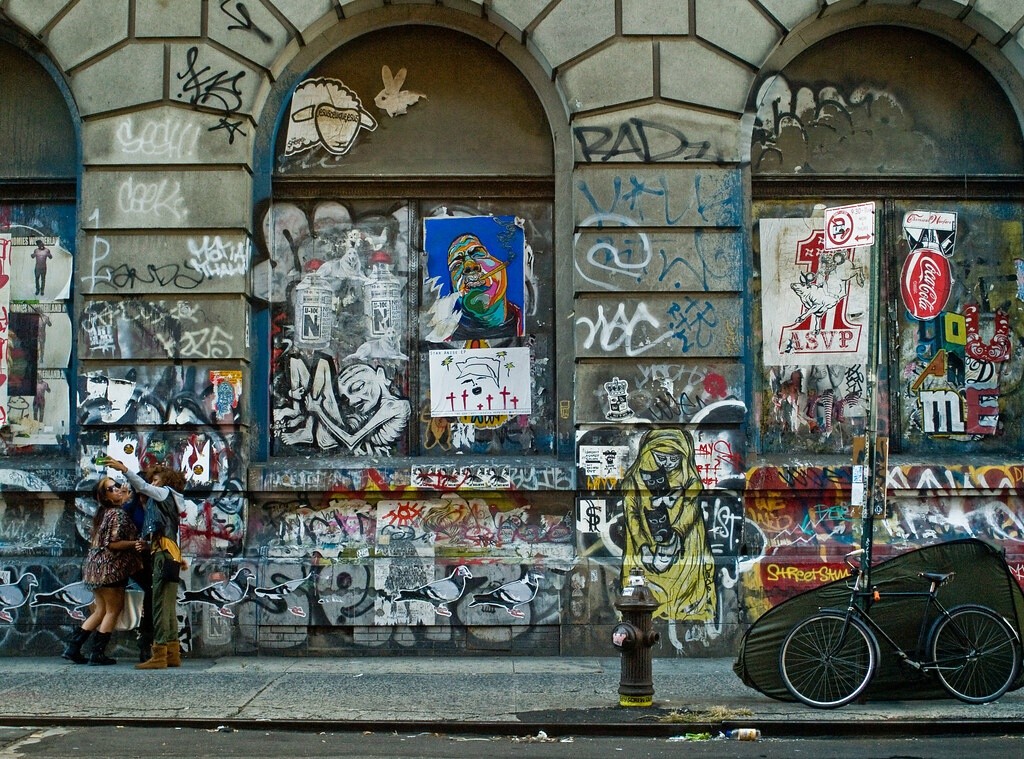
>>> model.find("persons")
[60,477,144,666]
[102,455,187,669]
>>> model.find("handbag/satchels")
[163,559,180,582]
[89,589,144,631]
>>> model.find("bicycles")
[780,546,1023,710]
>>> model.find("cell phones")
[96,457,110,465]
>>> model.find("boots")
[87,631,116,666]
[136,645,168,668]
[138,608,154,661]
[62,626,89,664]
[167,641,180,666]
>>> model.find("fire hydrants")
[610,567,660,706]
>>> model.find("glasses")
[105,483,121,492]
